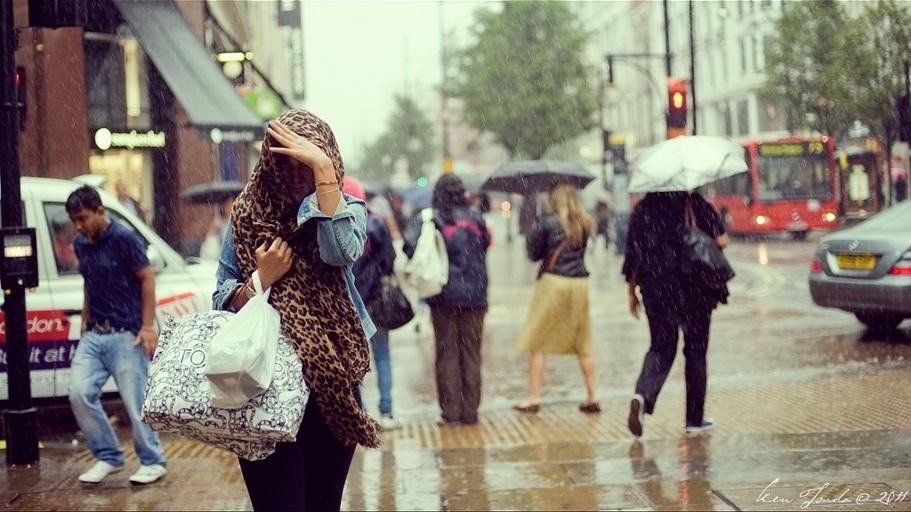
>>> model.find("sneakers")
[437,415,461,426]
[77,460,127,484]
[628,394,645,437]
[128,462,168,484]
[381,412,397,431]
[686,417,715,433]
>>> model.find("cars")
[809,197,911,328]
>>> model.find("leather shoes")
[513,403,539,412]
[579,400,600,412]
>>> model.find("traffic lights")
[668,83,688,128]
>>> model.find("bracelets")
[316,180,340,197]
[142,326,155,333]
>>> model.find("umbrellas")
[478,160,596,201]
[620,134,750,195]
[177,180,254,206]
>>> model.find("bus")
[631,136,840,244]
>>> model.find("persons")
[875,151,908,209]
[199,194,237,259]
[111,180,177,246]
[211,110,384,512]
[620,193,738,439]
[338,181,610,333]
[510,184,601,415]
[340,178,404,430]
[65,183,166,487]
[400,172,492,428]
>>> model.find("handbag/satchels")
[380,280,414,328]
[403,221,449,300]
[678,226,735,286]
[139,309,310,463]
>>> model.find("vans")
[0,176,215,401]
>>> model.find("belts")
[90,319,128,334]
